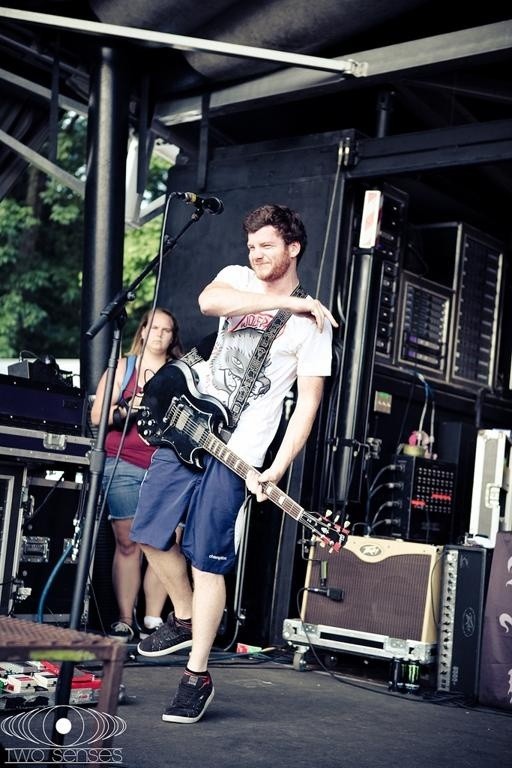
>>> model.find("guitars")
[136,358,351,555]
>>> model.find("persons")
[137,205,339,724]
[91,308,186,643]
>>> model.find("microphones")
[180,191,225,215]
[307,587,345,602]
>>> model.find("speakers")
[301,533,444,644]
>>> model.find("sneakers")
[162,673,214,723]
[108,612,193,656]
